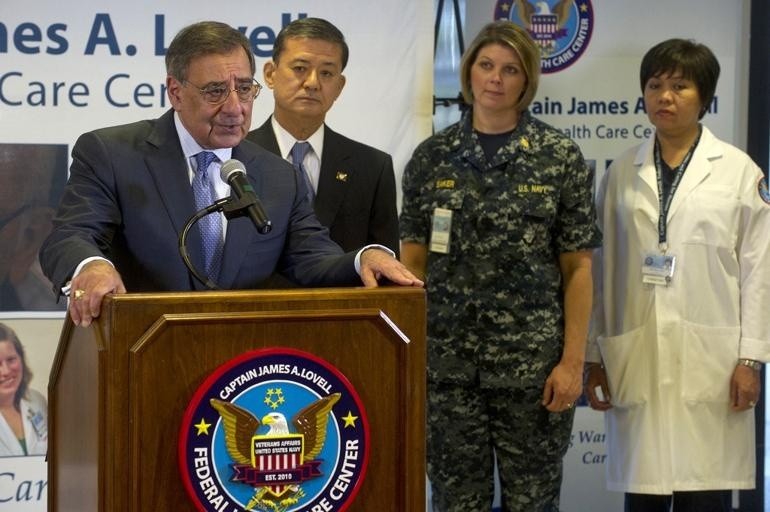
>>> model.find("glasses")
[180,78,262,105]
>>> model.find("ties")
[191,152,225,291]
[293,143,316,200]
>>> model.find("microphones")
[220,156,275,235]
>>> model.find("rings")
[72,289,88,300]
[566,402,571,407]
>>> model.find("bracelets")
[583,363,604,372]
[737,359,764,371]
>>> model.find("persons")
[664,256,672,270]
[38,21,425,329]
[391,22,600,511]
[581,36,768,509]
[1,323,51,458]
[246,18,402,286]
